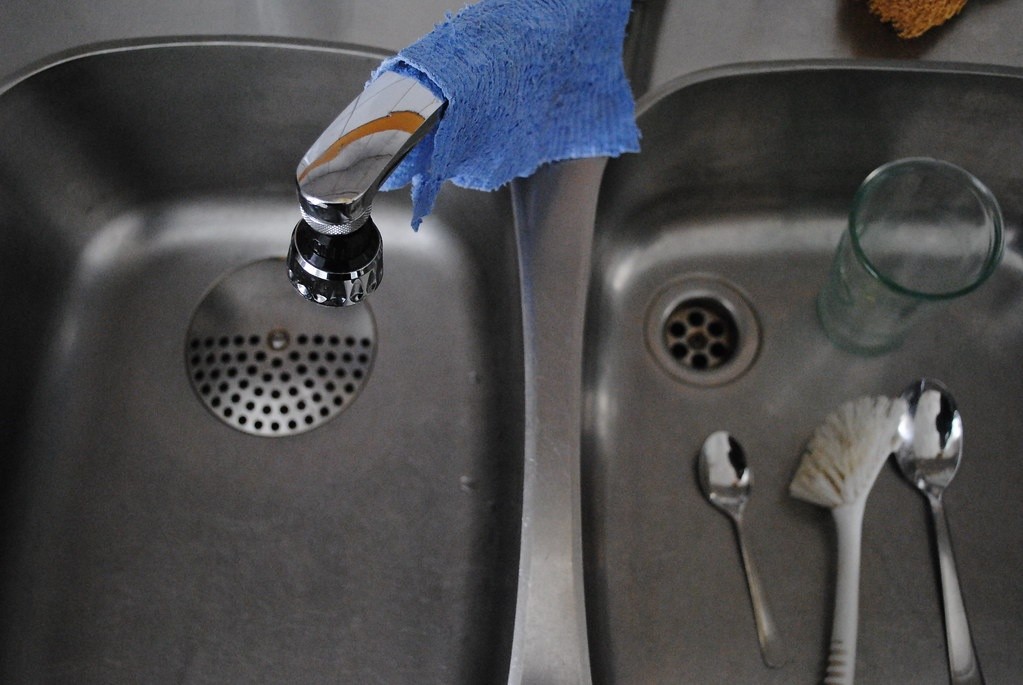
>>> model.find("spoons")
[891,374,991,685]
[700,426,792,669]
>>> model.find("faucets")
[286,59,448,306]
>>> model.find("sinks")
[0,36,526,685]
[578,58,1023,683]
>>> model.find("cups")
[818,154,1004,360]
